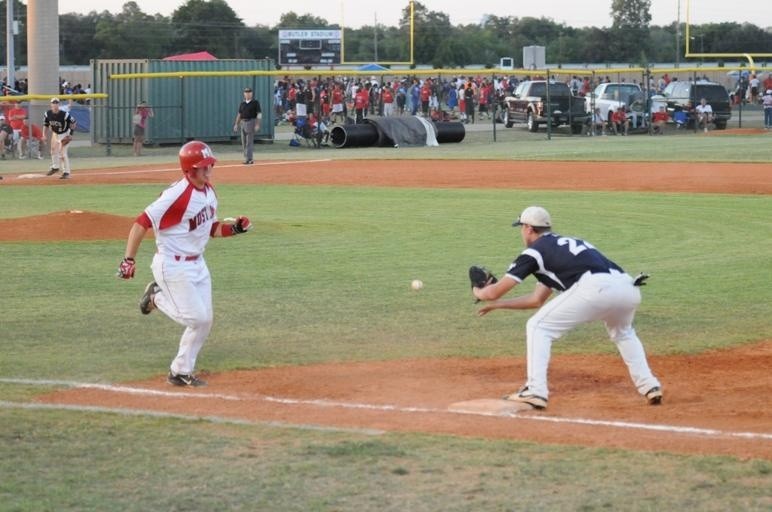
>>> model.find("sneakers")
[242,160,254,164]
[60,173,69,179]
[47,169,58,175]
[140,281,162,314]
[501,389,548,409]
[646,387,662,406]
[167,368,208,389]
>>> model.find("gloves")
[232,216,250,234]
[117,257,136,280]
[633,273,650,286]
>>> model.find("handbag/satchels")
[132,114,142,124]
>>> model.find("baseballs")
[412,280,423,290]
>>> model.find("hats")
[511,206,552,227]
[50,98,60,103]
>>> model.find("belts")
[175,255,201,261]
[590,267,623,274]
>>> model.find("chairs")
[295,117,320,148]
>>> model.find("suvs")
[648,78,734,130]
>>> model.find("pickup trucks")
[499,78,588,135]
[582,82,668,134]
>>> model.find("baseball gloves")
[61,136,71,145]
[469,265,497,303]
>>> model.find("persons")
[117,141,251,388]
[272,70,772,146]
[466,205,664,407]
[0,73,91,180]
[132,101,155,156]
[233,87,262,165]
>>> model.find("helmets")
[178,141,217,172]
